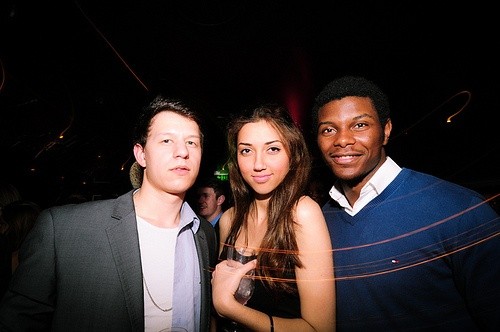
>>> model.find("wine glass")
[226,247,255,331]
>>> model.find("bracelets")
[268,315,274,332]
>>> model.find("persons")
[315,76,500,332]
[210,105,336,332]
[0,97,218,332]
[196,180,226,230]
